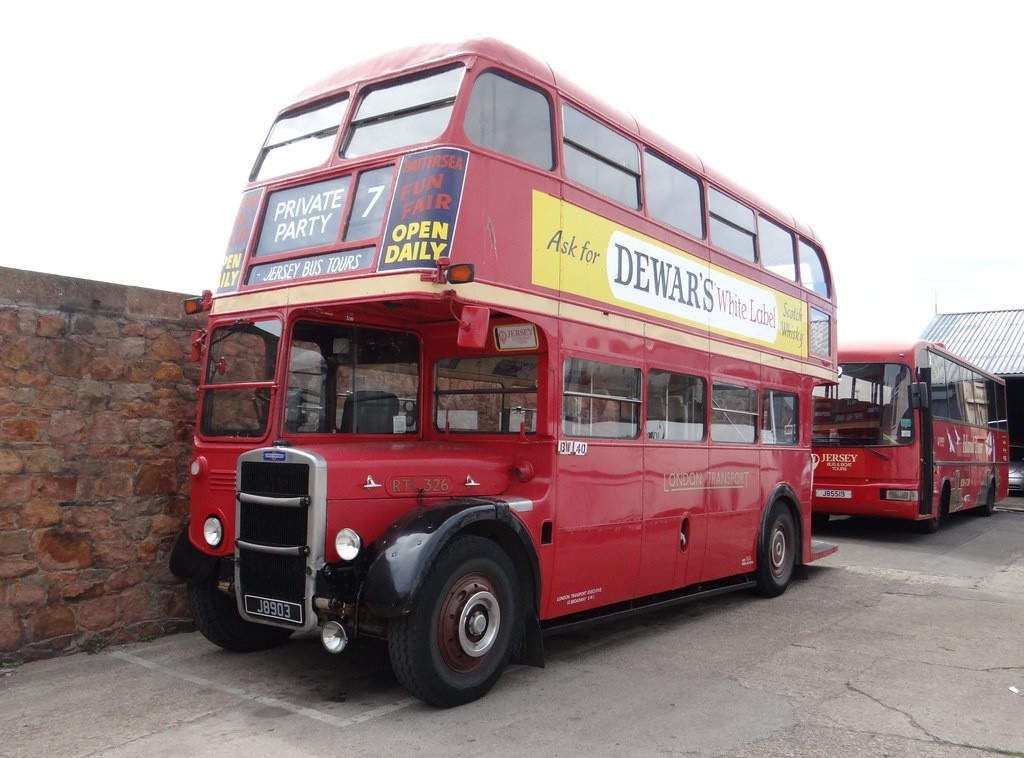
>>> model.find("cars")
[1008,441,1024,497]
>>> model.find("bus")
[810,333,1011,534]
[168,34,839,706]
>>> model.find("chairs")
[650,395,686,422]
[338,390,400,436]
